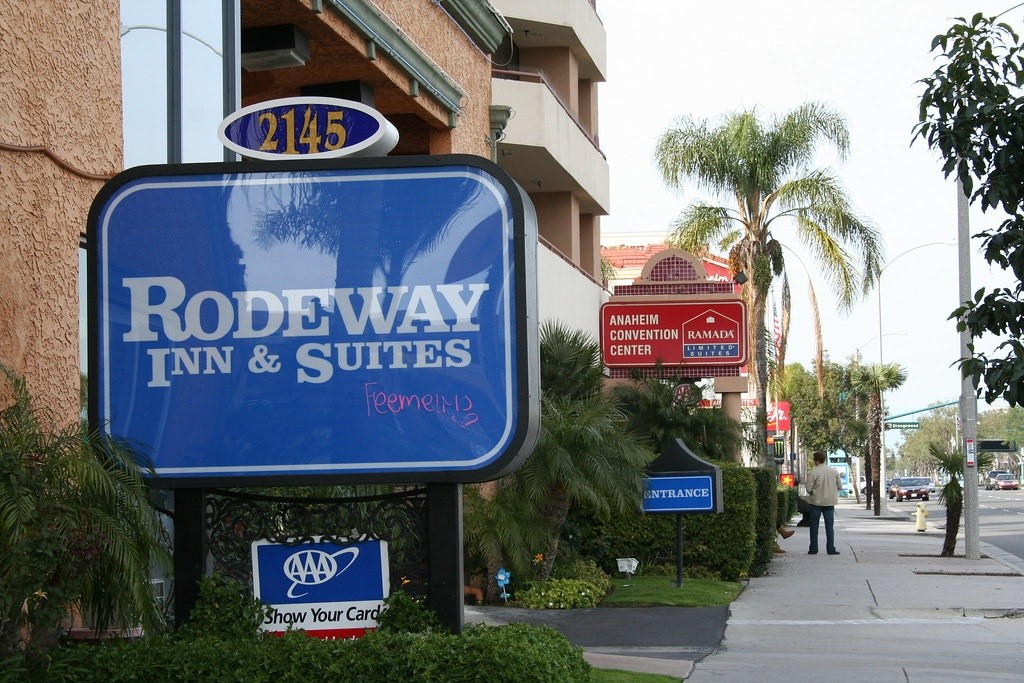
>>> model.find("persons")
[772,517,795,554]
[805,451,842,555]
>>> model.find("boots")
[778,527,794,539]
[774,545,786,553]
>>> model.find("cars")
[995,474,1019,490]
[895,477,930,502]
[849,476,874,495]
[886,477,901,499]
[929,481,936,492]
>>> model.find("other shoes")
[828,551,840,555]
[808,551,816,554]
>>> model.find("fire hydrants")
[911,502,930,532]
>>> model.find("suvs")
[984,470,1008,490]
